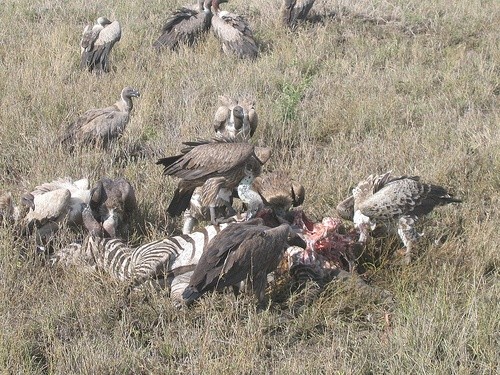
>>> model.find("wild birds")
[182,218,306,309]
[336,171,462,262]
[280,1,315,33]
[153,1,205,34]
[62,88,140,154]
[155,1,212,51]
[1,176,137,238]
[214,107,257,143]
[190,187,249,225]
[210,1,260,62]
[255,171,305,226]
[80,19,121,73]
[156,132,272,225]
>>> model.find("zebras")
[14,213,358,309]
